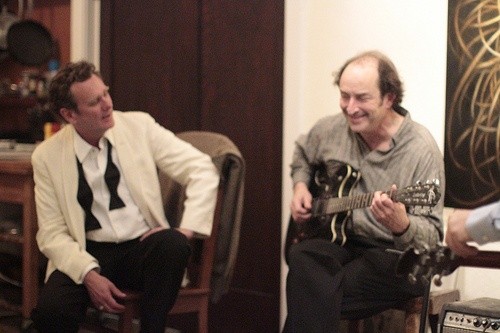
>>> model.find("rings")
[99,305,104,311]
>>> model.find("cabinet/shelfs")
[0,159,46,329]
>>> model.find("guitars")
[284,162,440,264]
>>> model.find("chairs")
[85,132,247,333]
[284,212,433,333]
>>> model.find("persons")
[445,200,500,258]
[31,60,220,333]
[283,52,445,333]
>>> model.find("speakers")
[436,296,500,333]
